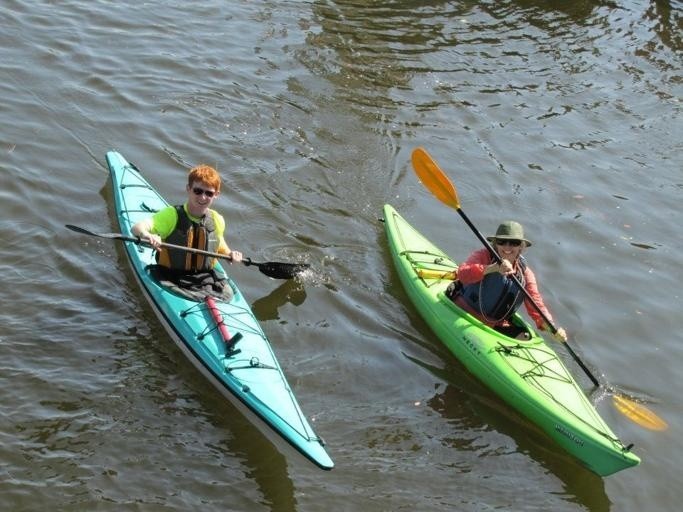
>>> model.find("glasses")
[496,239,520,247]
[192,186,215,197]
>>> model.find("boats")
[104,148,335,470]
[382,202,643,479]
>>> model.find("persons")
[452,220,566,342]
[131,164,242,272]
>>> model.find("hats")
[487,221,533,247]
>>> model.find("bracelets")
[139,232,147,237]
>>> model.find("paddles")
[65,224,311,279]
[412,147,668,432]
